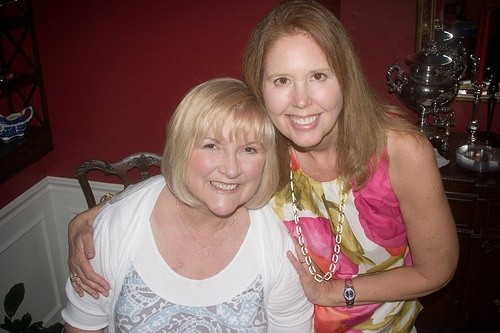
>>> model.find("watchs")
[343,277,356,309]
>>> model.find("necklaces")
[175,195,239,256]
[289,141,348,283]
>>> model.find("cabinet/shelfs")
[0,0,54,186]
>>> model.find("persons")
[59,79,315,333]
[68,1,459,333]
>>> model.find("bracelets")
[99,193,115,204]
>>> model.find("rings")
[69,274,77,282]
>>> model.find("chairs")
[75,151,161,209]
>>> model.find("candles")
[476,10,490,85]
[474,14,484,58]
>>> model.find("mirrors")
[415,0,500,103]
